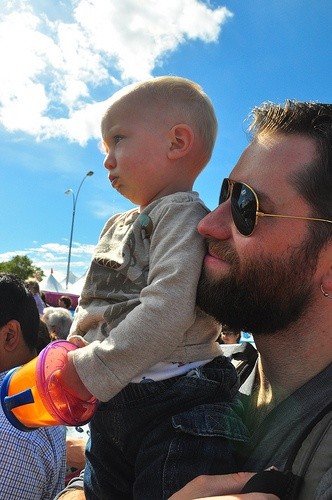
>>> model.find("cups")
[0,340,99,432]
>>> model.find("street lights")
[63,170,95,290]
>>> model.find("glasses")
[219,177,332,237]
[222,332,239,336]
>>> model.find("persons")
[60,76,252,500]
[1,270,68,500]
[60,100,332,500]
[25,277,75,341]
[221,324,250,380]
